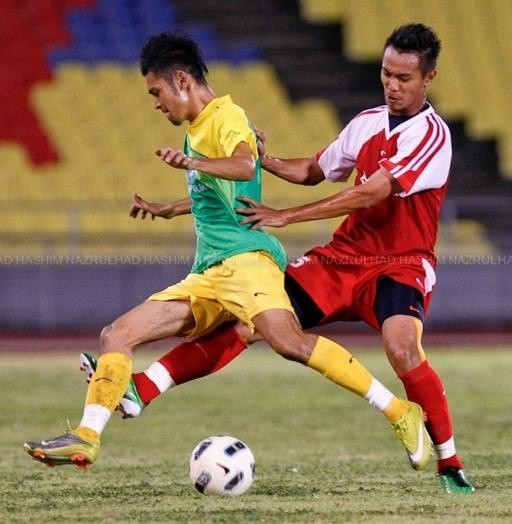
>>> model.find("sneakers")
[23,419,100,469]
[437,466,475,494]
[391,401,431,470]
[79,352,146,419]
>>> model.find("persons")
[22,29,433,471]
[231,20,477,497]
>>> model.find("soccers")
[189,436,255,496]
